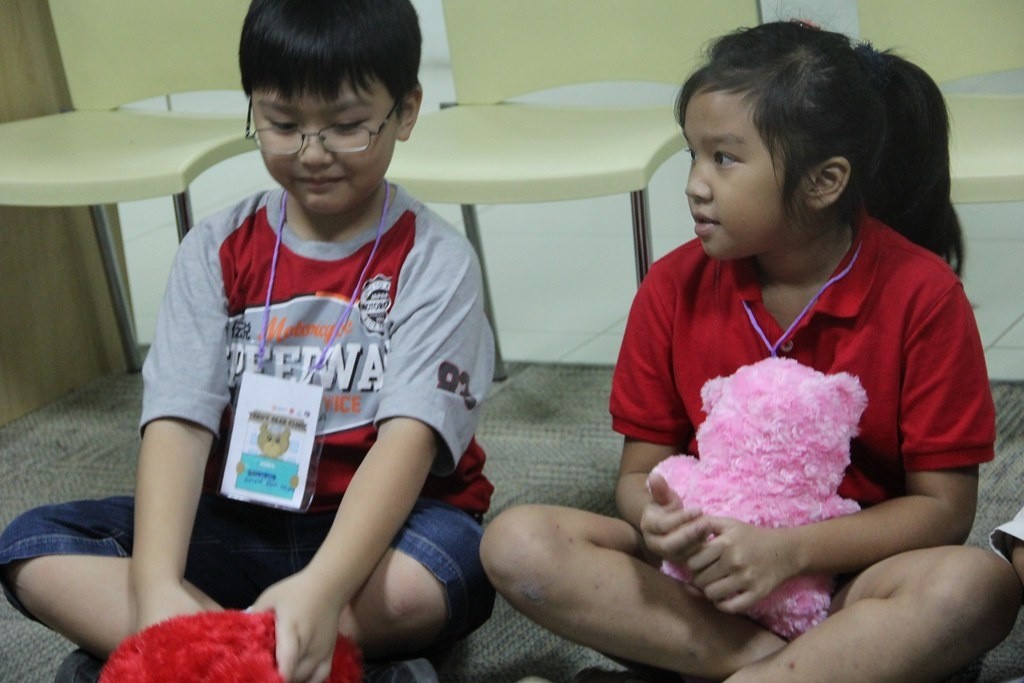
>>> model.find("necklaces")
[743,236,863,355]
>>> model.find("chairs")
[856,2,1022,205]
[381,1,762,383]
[0,0,258,377]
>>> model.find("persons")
[479,18,1024,683]
[0,0,497,683]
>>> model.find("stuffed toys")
[645,356,865,639]
[100,608,363,683]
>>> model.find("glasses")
[245,96,399,155]
